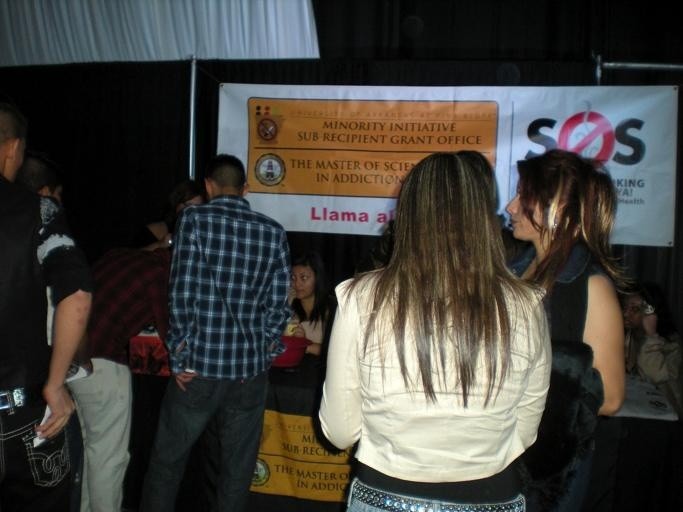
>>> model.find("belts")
[0,388,26,410]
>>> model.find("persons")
[316,149,556,511]
[457,148,537,278]
[503,150,629,510]
[285,253,334,356]
[620,283,682,386]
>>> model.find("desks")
[121,369,682,512]
